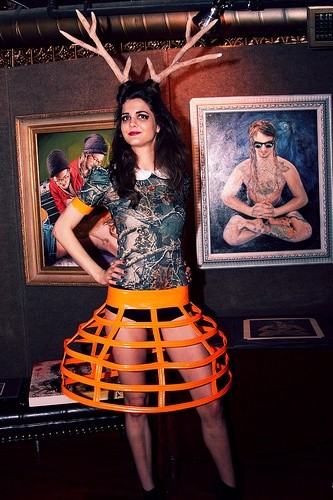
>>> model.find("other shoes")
[214,475,242,499]
[144,487,165,500]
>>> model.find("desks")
[0,378,180,477]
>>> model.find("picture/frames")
[190,94,331,270]
[14,107,117,285]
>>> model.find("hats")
[82,133,107,154]
[47,150,70,177]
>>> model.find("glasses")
[91,154,101,166]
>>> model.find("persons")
[52,79,243,500]
